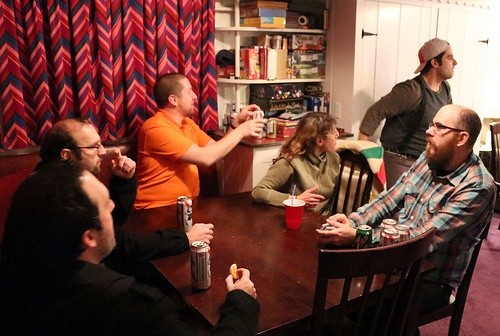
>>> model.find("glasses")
[428,122,471,139]
[69,142,102,153]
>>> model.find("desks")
[129,191,399,336]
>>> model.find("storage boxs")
[240,0,331,138]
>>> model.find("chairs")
[320,123,500,336]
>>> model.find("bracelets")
[230,123,236,129]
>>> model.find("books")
[305,90,330,113]
[253,35,325,79]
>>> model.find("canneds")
[266,119,277,138]
[355,224,372,249]
[176,196,193,232]
[379,219,410,246]
[190,240,211,290]
[252,110,264,138]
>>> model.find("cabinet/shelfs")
[214,0,353,196]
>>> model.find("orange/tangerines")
[229,264,239,279]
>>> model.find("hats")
[414,38,451,74]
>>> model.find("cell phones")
[318,223,331,238]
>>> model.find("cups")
[283,199,306,230]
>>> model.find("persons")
[252,111,343,216]
[357,38,457,191]
[0,158,260,336]
[133,73,266,208]
[315,103,497,336]
[0,120,214,267]
